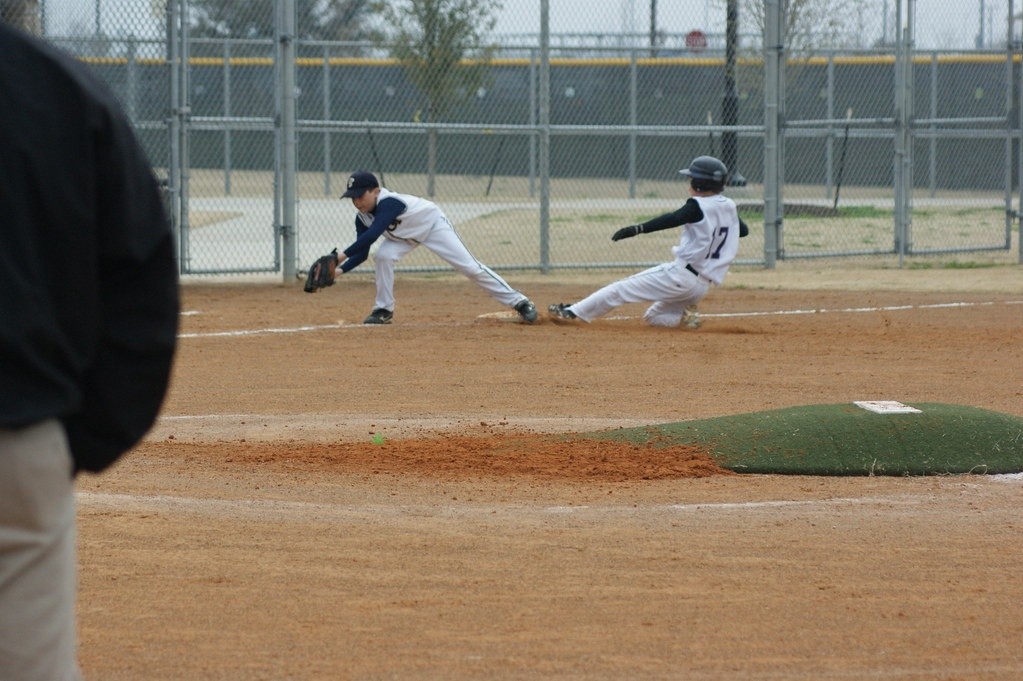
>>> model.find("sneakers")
[515,300,537,322]
[363,308,393,324]
[547,303,576,321]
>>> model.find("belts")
[686,264,712,283]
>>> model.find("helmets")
[678,156,728,181]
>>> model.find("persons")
[0,1,183,681]
[546,155,749,326]
[304,170,538,321]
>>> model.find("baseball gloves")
[303,247,339,294]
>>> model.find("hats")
[340,170,378,200]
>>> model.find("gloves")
[612,224,641,242]
